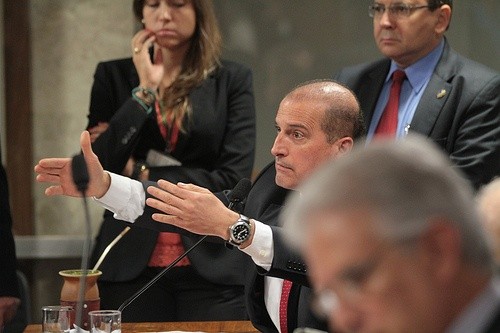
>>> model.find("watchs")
[225,214,252,250]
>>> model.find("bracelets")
[129,161,147,181]
[132,86,156,114]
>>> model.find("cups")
[88,310,121,333]
[42,305,72,333]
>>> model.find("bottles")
[58,268,102,331]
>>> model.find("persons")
[337,0,500,194]
[81,0,255,323]
[34,79,361,333]
[276,133,500,333]
[0,159,29,333]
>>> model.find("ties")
[279,279,293,333]
[370,69,406,147]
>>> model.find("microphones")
[118,178,252,311]
[70,155,94,329]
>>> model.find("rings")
[134,47,141,54]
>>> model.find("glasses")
[310,250,389,317]
[368,3,434,17]
